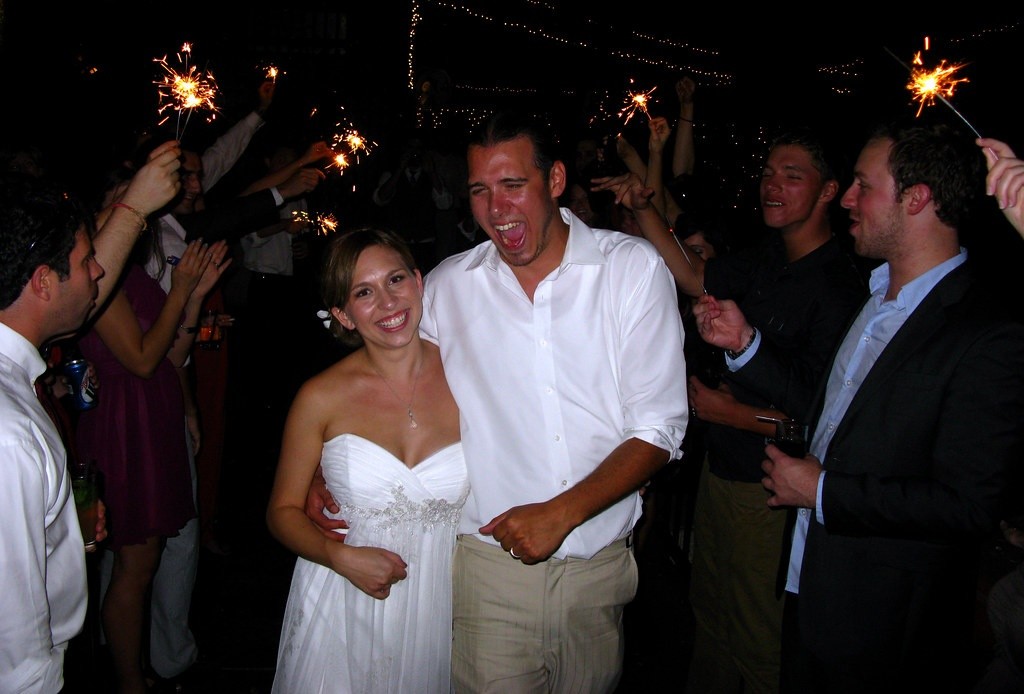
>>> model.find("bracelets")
[680,114,694,123]
[112,202,147,230]
[178,322,197,334]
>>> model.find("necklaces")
[367,342,424,429]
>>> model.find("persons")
[306,109,689,694]
[0,82,491,694]
[558,77,1024,694]
[267,229,471,694]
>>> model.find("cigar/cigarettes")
[222,317,235,323]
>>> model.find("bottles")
[72,462,98,546]
[294,231,306,272]
[775,419,808,460]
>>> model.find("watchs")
[726,323,756,359]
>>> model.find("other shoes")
[141,667,181,694]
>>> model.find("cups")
[200,309,222,352]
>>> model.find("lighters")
[166,254,180,265]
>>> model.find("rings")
[213,261,218,267]
[510,547,521,558]
[691,407,697,418]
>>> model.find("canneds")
[63,359,98,410]
